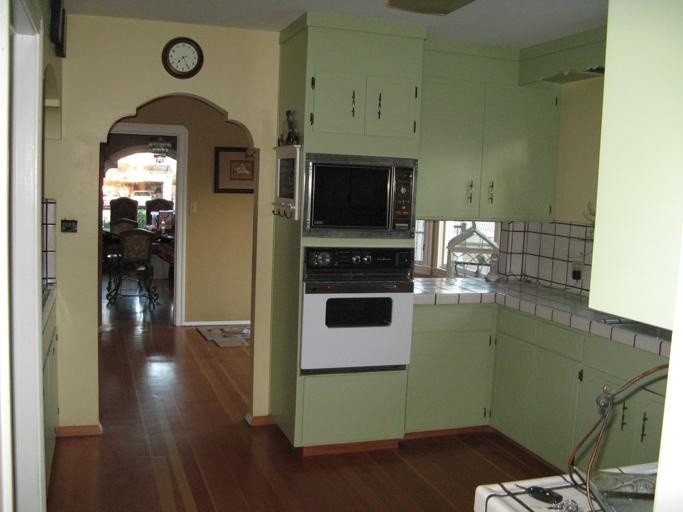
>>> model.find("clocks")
[161,34,205,79]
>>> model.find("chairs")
[97,196,175,308]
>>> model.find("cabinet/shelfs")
[310,68,418,136]
[553,81,602,220]
[417,72,552,220]
[491,307,586,473]
[405,305,497,433]
[573,334,670,493]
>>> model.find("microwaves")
[303,153,417,238]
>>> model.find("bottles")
[161,219,166,234]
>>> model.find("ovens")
[300,246,413,373]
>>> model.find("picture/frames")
[214,145,256,194]
